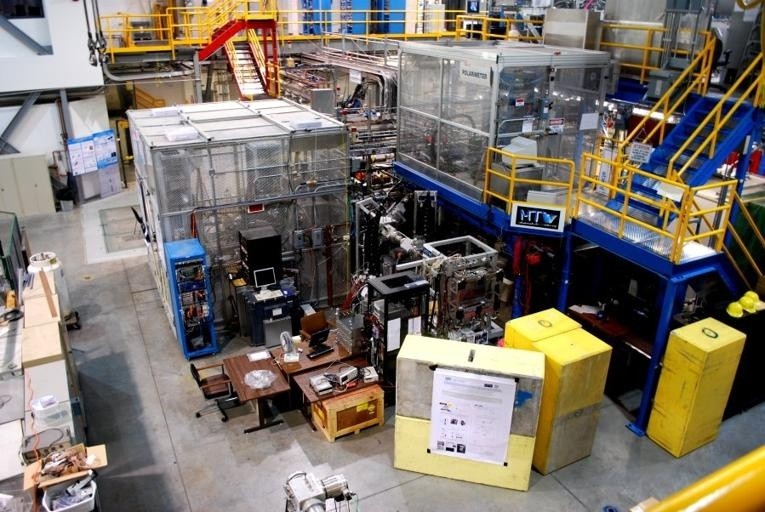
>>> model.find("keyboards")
[255,289,283,301]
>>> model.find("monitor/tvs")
[309,328,331,352]
[253,267,276,294]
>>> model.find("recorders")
[337,366,359,385]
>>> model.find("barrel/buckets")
[26,250,73,320]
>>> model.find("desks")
[223,330,391,433]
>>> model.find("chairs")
[190,362,239,423]
[299,310,337,340]
